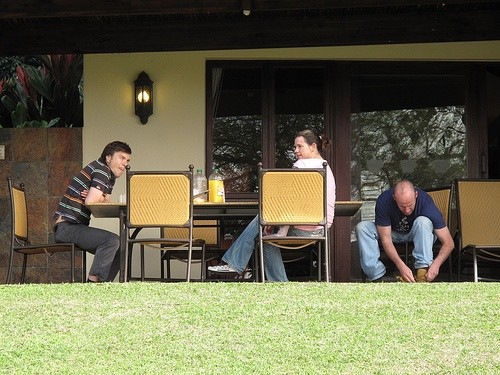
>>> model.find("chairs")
[125,164,205,283]
[254,162,328,283]
[361,179,455,284]
[456,178,500,282]
[158,219,226,283]
[6,177,86,285]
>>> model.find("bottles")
[193,168,207,204]
[208,168,226,203]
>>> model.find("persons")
[355,180,454,283]
[208,129,335,282]
[52,141,132,282]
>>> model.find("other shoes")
[415,268,427,282]
[208,261,237,273]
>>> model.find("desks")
[84,201,363,281]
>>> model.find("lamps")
[133,71,154,125]
